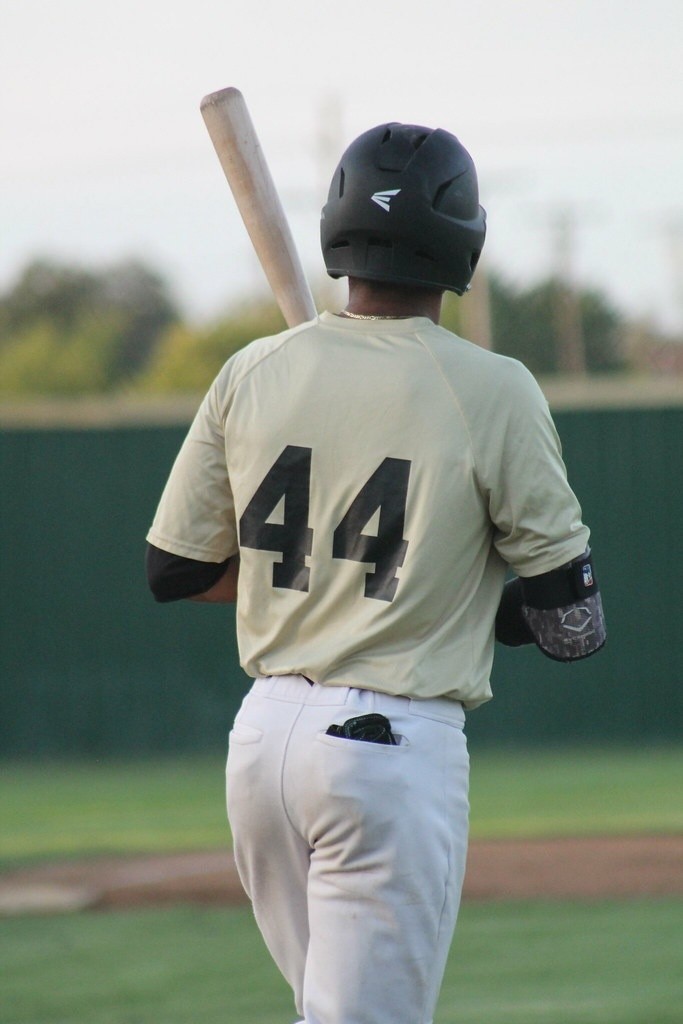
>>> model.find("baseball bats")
[197,83,317,326]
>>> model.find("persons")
[145,121,609,1024]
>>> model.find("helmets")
[320,123,487,296]
